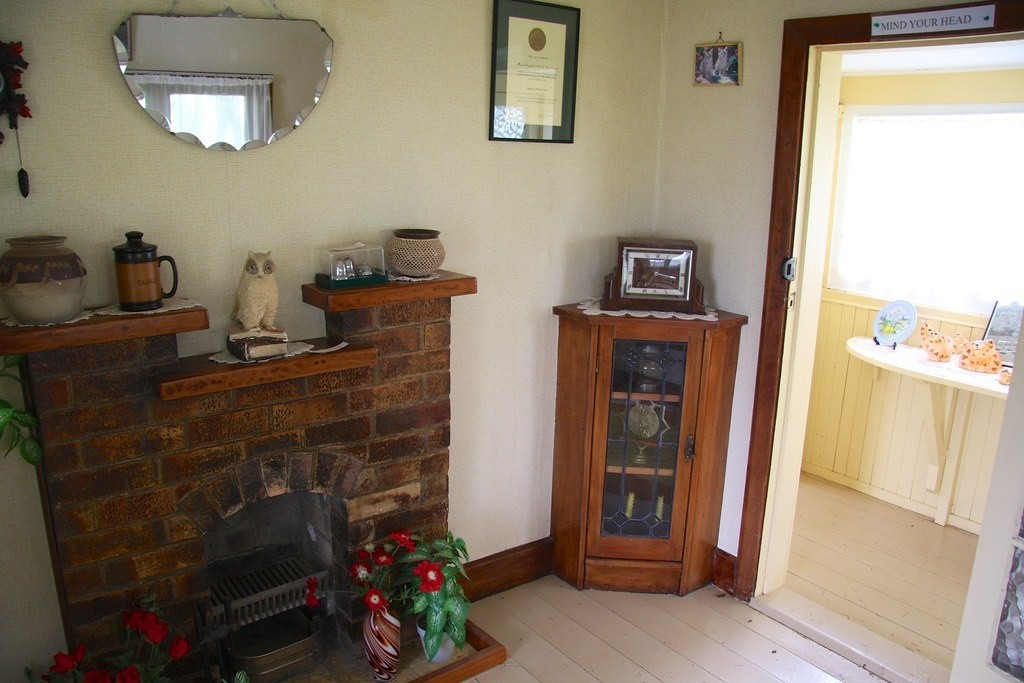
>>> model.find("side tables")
[550,301,749,597]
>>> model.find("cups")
[111,230,179,311]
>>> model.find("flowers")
[23,606,189,683]
[302,532,445,611]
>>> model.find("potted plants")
[396,533,473,662]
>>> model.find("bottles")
[1,235,87,324]
[386,228,445,278]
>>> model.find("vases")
[363,602,402,683]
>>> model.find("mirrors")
[113,13,335,153]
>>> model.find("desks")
[846,335,1013,526]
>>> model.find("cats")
[699,48,714,78]
[715,46,728,76]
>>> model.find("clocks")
[598,236,707,315]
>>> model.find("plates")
[873,301,917,345]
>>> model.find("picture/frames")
[693,41,745,87]
[489,0,583,145]
[983,300,1024,368]
